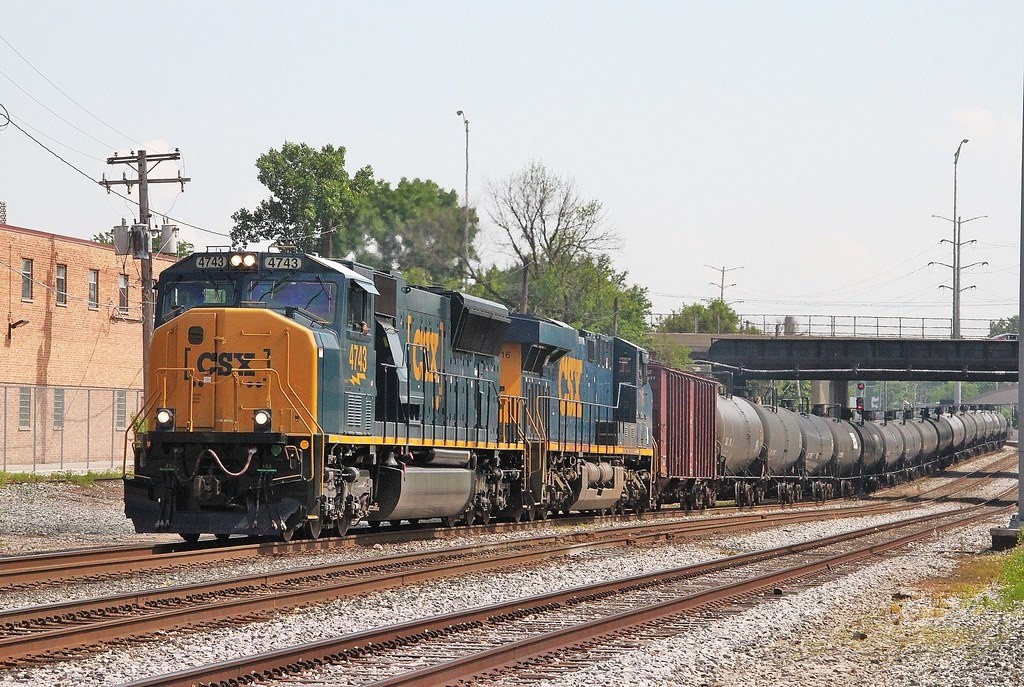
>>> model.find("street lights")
[951,139,970,341]
[456,109,469,294]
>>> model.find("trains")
[119,244,1011,545]
